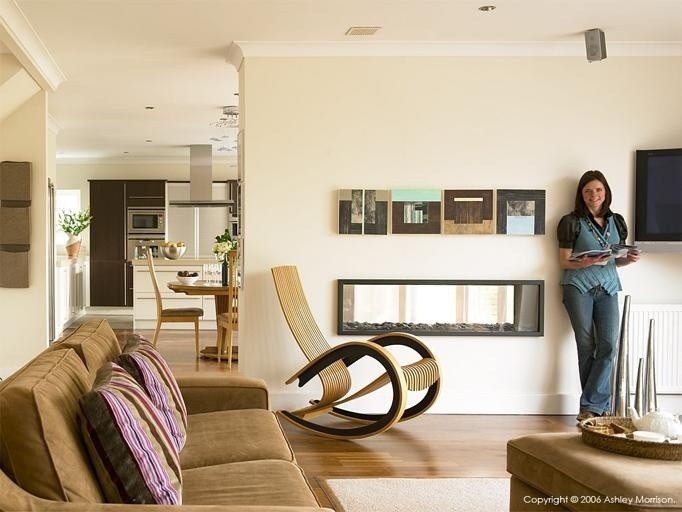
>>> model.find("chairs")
[217,249,241,371]
[271,265,441,441]
[144,249,208,359]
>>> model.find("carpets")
[311,475,511,512]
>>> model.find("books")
[568,244,642,266]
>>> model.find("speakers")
[585,28,607,63]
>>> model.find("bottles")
[222,253,229,286]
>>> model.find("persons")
[557,170,642,421]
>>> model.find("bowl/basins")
[176,276,199,285]
[161,246,187,260]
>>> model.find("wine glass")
[203,263,221,287]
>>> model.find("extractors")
[169,145,235,208]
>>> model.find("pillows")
[120,334,187,455]
[79,361,181,505]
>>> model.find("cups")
[632,431,670,443]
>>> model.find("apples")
[161,241,187,247]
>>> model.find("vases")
[219,263,242,286]
[67,237,82,258]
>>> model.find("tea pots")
[627,407,682,438]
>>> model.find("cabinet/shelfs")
[132,256,204,330]
[204,258,240,321]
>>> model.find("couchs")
[2,317,323,512]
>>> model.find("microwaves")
[127,240,165,261]
[127,210,165,234]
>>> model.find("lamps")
[211,105,239,129]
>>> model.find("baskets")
[578,416,682,461]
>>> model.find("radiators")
[618,306,682,398]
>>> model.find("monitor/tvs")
[634,148,682,253]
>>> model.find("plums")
[178,270,198,276]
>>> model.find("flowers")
[56,207,95,237]
[212,229,237,263]
